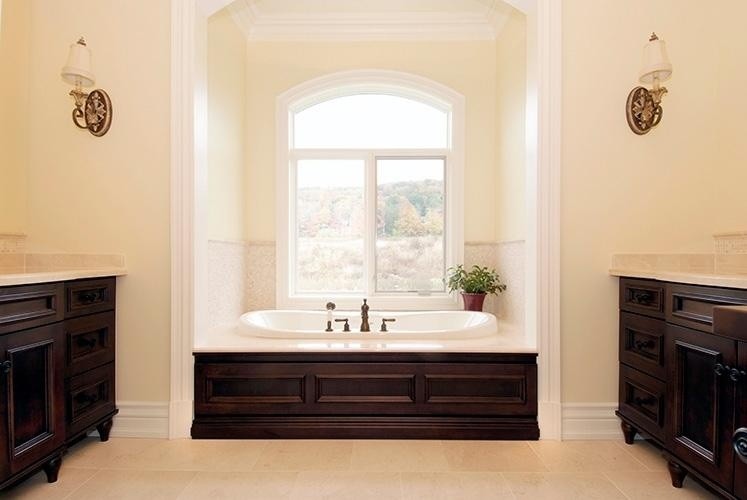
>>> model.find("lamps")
[626,32,672,135]
[60,35,112,137]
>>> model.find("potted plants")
[442,264,507,312]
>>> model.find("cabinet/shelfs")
[615,276,747,500]
[0,276,119,496]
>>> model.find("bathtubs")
[240,307,498,338]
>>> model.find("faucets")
[360,299,370,332]
